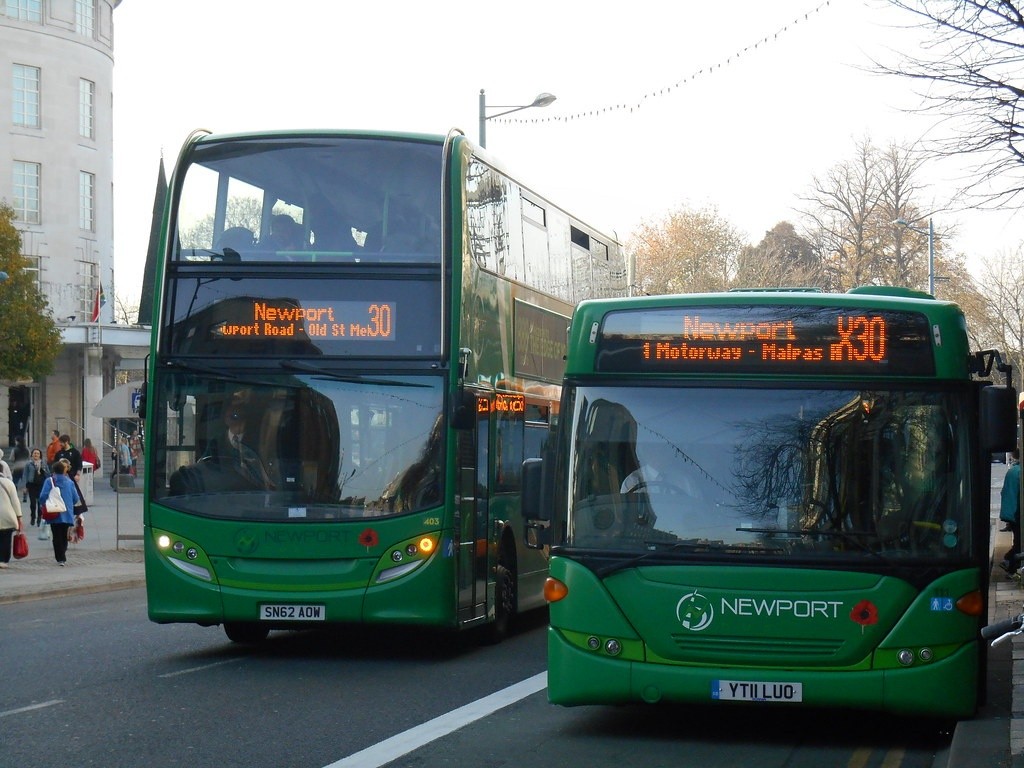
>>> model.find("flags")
[90,285,106,323]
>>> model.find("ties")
[231,436,242,472]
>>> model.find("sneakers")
[58,561,66,566]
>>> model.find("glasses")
[226,413,247,419]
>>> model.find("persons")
[0,435,29,570]
[201,402,264,471]
[21,429,102,567]
[111,430,145,480]
[620,434,703,502]
[1000,448,1024,580]
[306,176,421,252]
[266,212,306,262]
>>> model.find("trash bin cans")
[78,461,94,507]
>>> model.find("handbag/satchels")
[41,477,59,521]
[13,522,28,559]
[96,456,101,468]
[32,460,45,485]
[37,519,48,540]
[67,514,84,544]
[45,477,66,512]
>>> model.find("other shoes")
[0,562,8,568]
[1006,572,1021,580]
[999,559,1010,572]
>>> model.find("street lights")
[892,218,934,297]
[479,89,557,149]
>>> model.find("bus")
[521,285,1019,748]
[139,130,630,646]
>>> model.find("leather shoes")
[1000,524,1011,531]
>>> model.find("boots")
[30,507,36,525]
[22,491,27,502]
[37,509,41,527]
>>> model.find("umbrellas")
[91,381,146,418]
